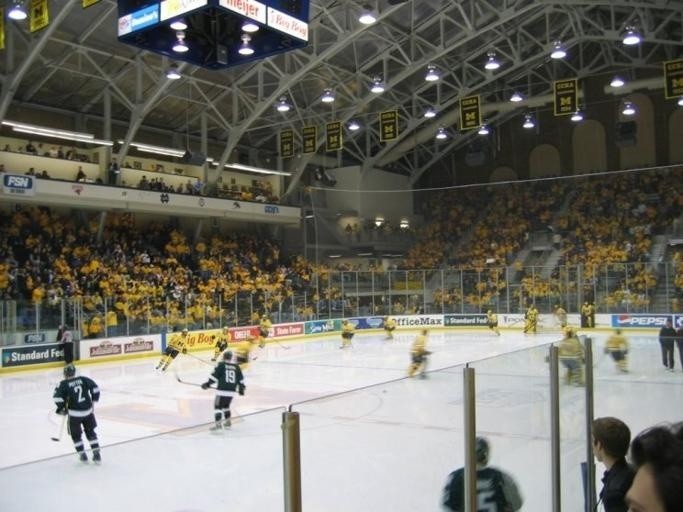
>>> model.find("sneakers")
[81,453,87,461]
[224,420,231,426]
[211,424,222,431]
[93,452,100,460]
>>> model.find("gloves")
[239,387,244,395]
[202,383,209,389]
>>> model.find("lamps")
[7,0,27,20]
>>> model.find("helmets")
[224,351,232,359]
[64,365,75,376]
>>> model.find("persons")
[442,437,522,512]
[1,138,683,388]
[623,421,683,512]
[53,365,101,461]
[202,351,245,430]
[592,417,636,512]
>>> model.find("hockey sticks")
[175,374,217,390]
[269,336,292,350]
[247,356,258,360]
[187,351,218,368]
[51,415,67,442]
